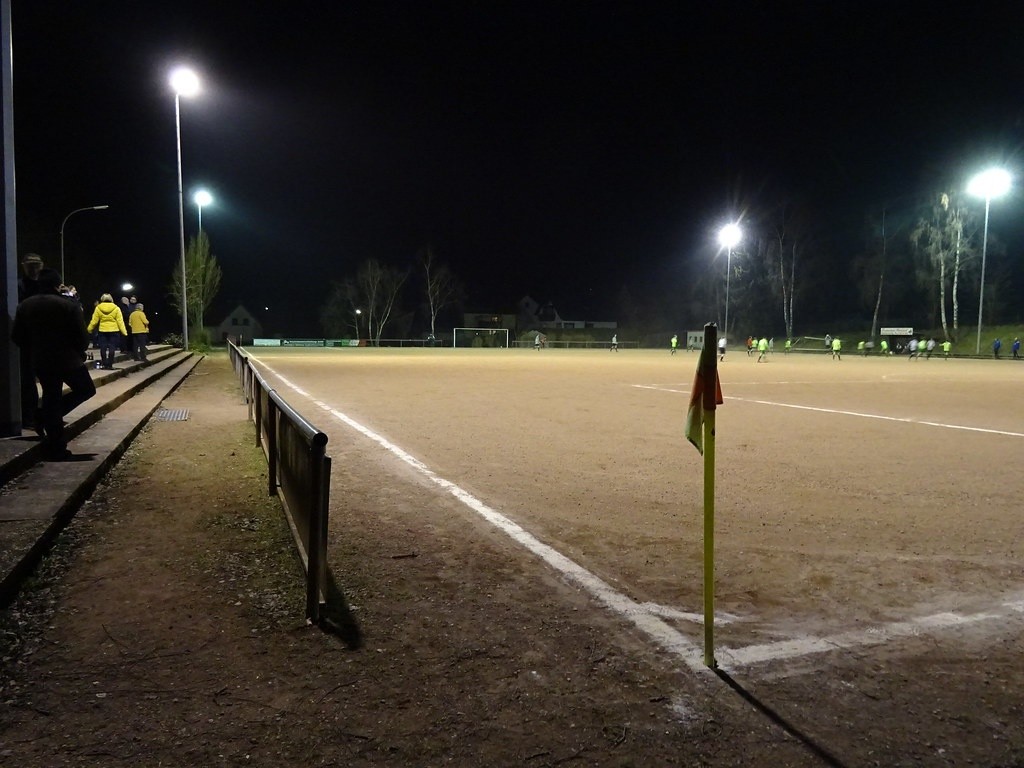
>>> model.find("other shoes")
[141,357,146,360]
[103,365,108,369]
[108,366,114,369]
[23,422,35,429]
[30,409,46,437]
[47,450,71,461]
[134,357,140,361]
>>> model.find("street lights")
[355,309,362,339]
[171,66,197,349]
[718,223,743,338]
[971,167,1013,352]
[193,188,212,328]
[60,206,110,285]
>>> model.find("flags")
[685,333,724,455]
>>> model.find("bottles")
[96,362,99,369]
[87,352,93,360]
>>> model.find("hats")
[22,255,43,265]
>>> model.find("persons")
[540,337,546,348]
[87,292,150,370]
[747,336,774,363]
[992,338,1000,359]
[1011,337,1020,358]
[58,283,83,310]
[17,252,46,428]
[15,270,96,460]
[825,334,831,354]
[609,334,619,352]
[533,334,540,351]
[718,336,727,361]
[670,335,678,355]
[784,337,791,355]
[687,336,693,352]
[906,337,951,360]
[857,339,909,356]
[832,336,841,360]
